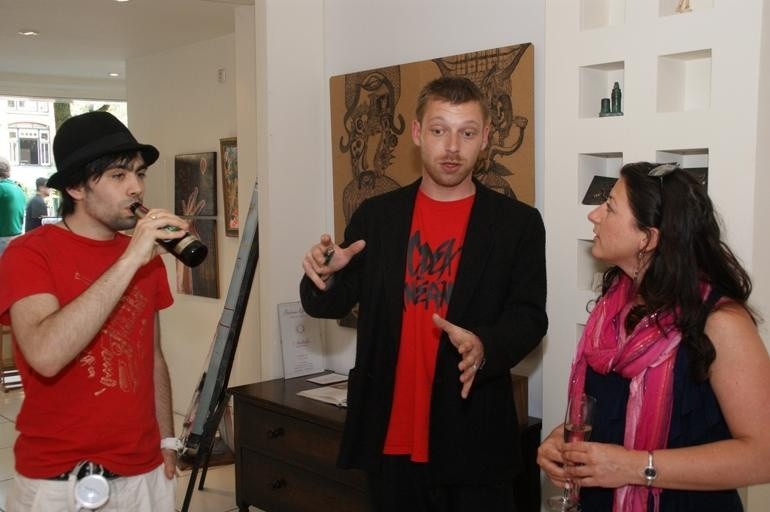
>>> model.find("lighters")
[324,247,335,266]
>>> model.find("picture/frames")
[219,137,239,238]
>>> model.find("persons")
[0,160,26,253]
[534,159,770,512]
[300,75,548,511]
[25,177,51,233]
[2,111,185,511]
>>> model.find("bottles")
[611,82,622,112]
[131,203,208,268]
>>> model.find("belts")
[48,459,119,481]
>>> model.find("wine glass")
[545,394,598,511]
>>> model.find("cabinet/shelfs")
[543,0,762,442]
[226,370,542,512]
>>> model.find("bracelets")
[161,437,185,457]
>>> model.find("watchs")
[641,447,657,485]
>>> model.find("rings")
[151,214,156,219]
[471,364,480,375]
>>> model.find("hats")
[45,111,160,189]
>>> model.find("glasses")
[647,162,681,205]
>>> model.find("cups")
[601,98,610,114]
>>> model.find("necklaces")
[63,218,72,231]
